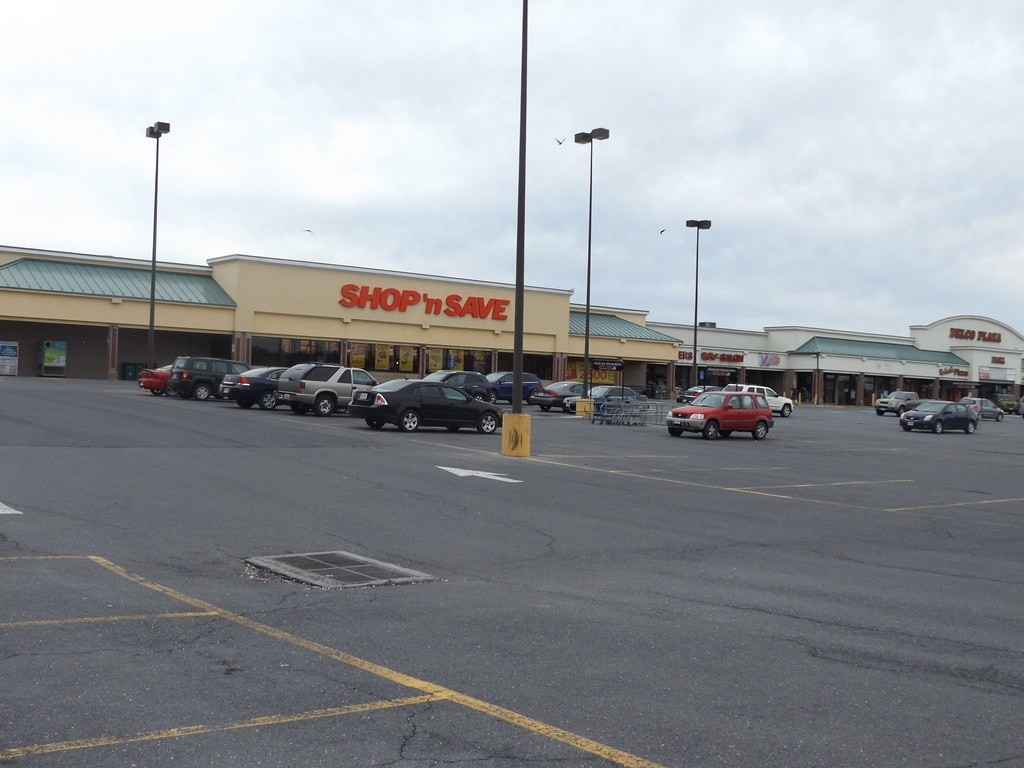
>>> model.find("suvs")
[666,390,774,441]
[422,370,493,400]
[722,384,794,416]
[993,394,1019,415]
[167,355,252,401]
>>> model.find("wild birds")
[304,229,312,233]
[660,229,666,234]
[556,137,566,145]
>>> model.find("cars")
[958,397,1004,422]
[676,386,724,403]
[530,381,593,412]
[347,379,503,434]
[575,127,610,399]
[138,365,178,397]
[274,361,380,417]
[218,367,291,410]
[562,386,649,416]
[898,400,979,434]
[487,371,543,404]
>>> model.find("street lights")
[146,121,172,370]
[685,219,712,389]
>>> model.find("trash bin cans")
[123,362,145,380]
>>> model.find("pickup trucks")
[874,390,935,417]
